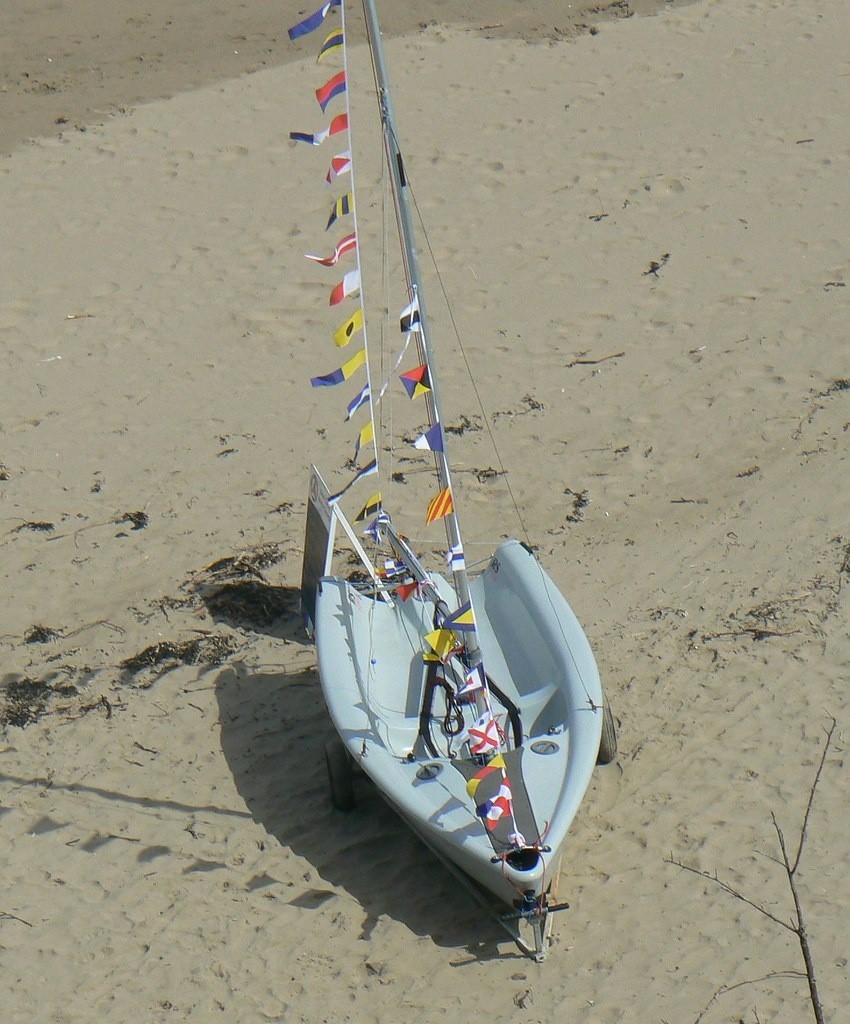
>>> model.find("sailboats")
[295,2,606,959]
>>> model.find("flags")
[332,308,363,348]
[314,71,346,114]
[304,232,356,267]
[364,509,394,546]
[329,270,360,306]
[316,28,343,64]
[344,383,370,422]
[287,0,342,41]
[327,458,378,507]
[310,349,367,389]
[352,422,374,466]
[383,293,525,832]
[290,113,347,146]
[325,192,354,231]
[324,149,351,188]
[351,492,383,526]
